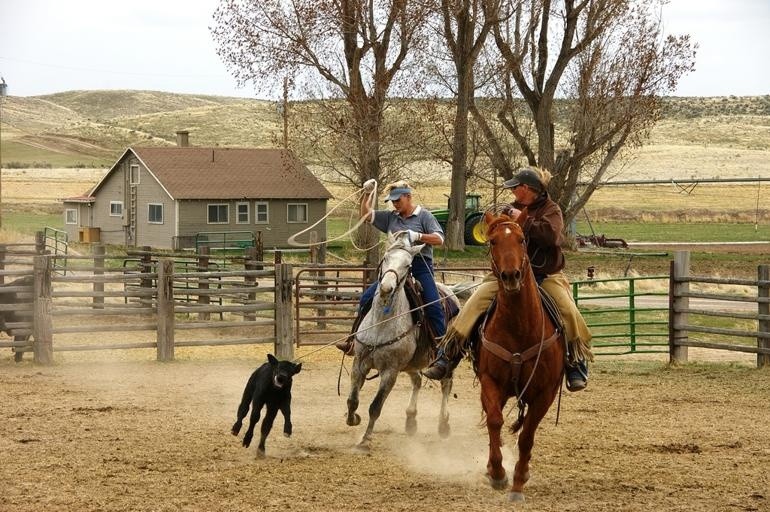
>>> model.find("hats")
[503,170,540,188]
[384,187,411,203]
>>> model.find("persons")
[336,180,446,355]
[423,170,587,391]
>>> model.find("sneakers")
[423,365,452,379]
[336,342,353,355]
[570,378,586,392]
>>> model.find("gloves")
[407,229,418,242]
[363,179,375,193]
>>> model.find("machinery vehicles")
[430,190,494,246]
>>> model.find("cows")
[229,352,303,460]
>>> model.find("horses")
[342,229,475,459]
[469,205,567,504]
